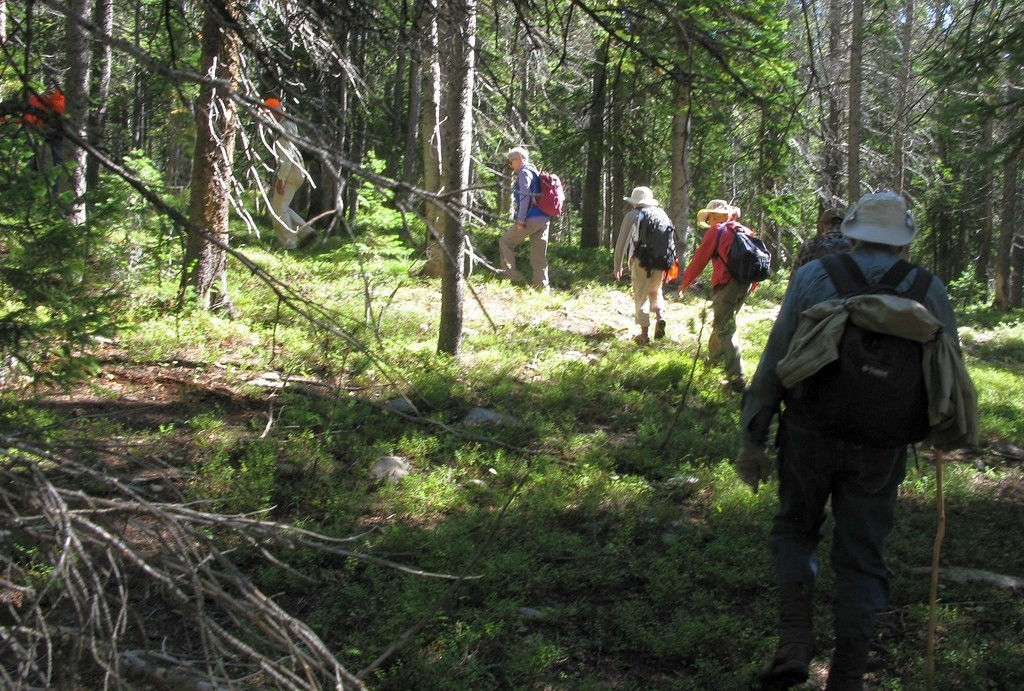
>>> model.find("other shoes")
[756,631,813,685]
[825,668,864,691]
[635,335,648,344]
[653,319,667,342]
[300,231,317,249]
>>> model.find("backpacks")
[520,167,565,216]
[631,208,675,275]
[781,250,933,452]
[715,226,770,283]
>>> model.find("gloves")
[733,448,772,493]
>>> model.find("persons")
[734,191,961,691]
[614,186,676,344]
[498,146,552,294]
[261,98,318,251]
[790,207,857,275]
[1,73,67,193]
[677,199,760,391]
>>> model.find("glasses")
[508,158,515,165]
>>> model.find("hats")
[696,199,741,228]
[816,199,847,220]
[840,192,916,246]
[265,99,282,111]
[623,187,659,206]
